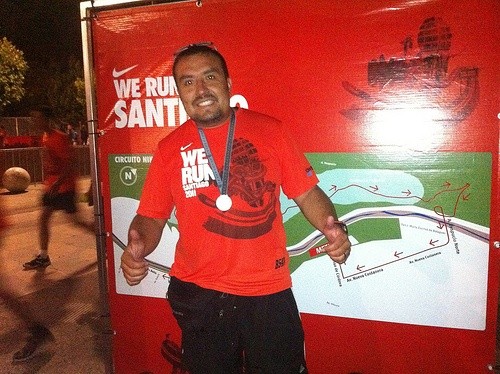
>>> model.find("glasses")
[174,42,217,59]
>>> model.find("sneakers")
[23,255,51,269]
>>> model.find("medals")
[215,193,233,212]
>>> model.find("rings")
[342,253,347,260]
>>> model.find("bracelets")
[330,219,348,233]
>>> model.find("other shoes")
[12,326,55,362]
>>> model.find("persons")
[22,104,95,268]
[116,44,351,374]
[0,119,89,150]
